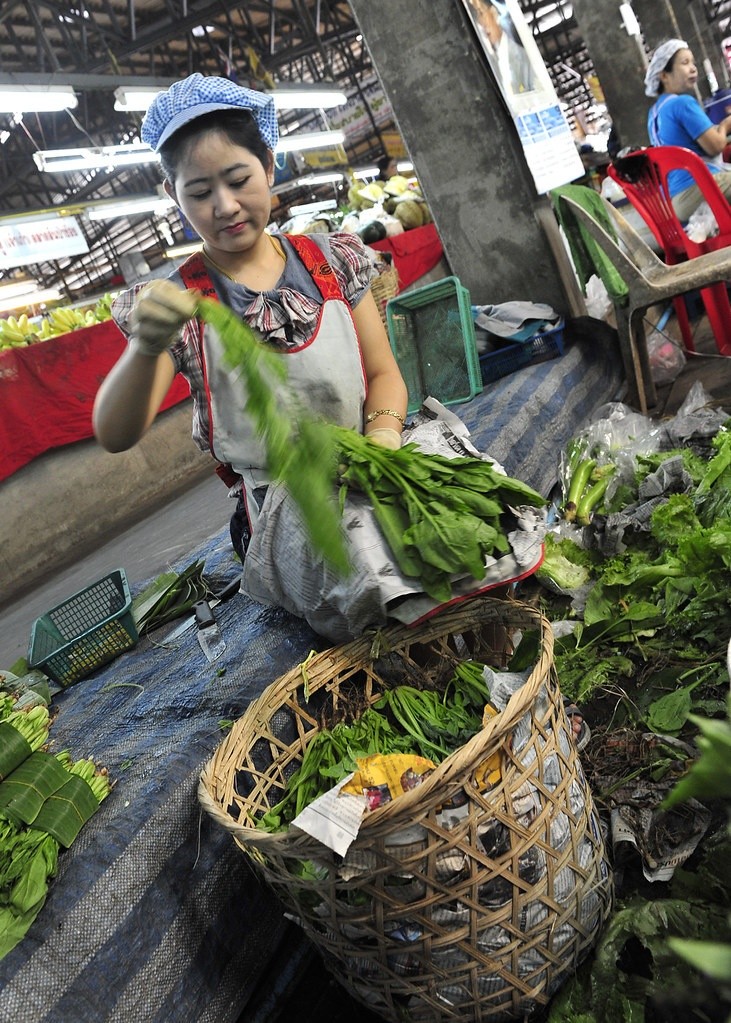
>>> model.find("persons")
[91,73,559,626]
[475,0,536,96]
[639,38,731,220]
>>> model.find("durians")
[348,175,431,228]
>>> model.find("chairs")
[551,182,730,418]
[607,146,731,359]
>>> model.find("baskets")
[370,267,398,341]
[196,593,616,1023]
[479,313,568,387]
[384,276,482,416]
[27,567,140,688]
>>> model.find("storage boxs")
[382,276,484,409]
[203,599,611,1022]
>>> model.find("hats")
[644,38,689,97]
[141,71,278,153]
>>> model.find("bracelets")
[365,406,405,425]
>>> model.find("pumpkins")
[362,220,388,244]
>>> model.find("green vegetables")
[0,296,731,1022]
